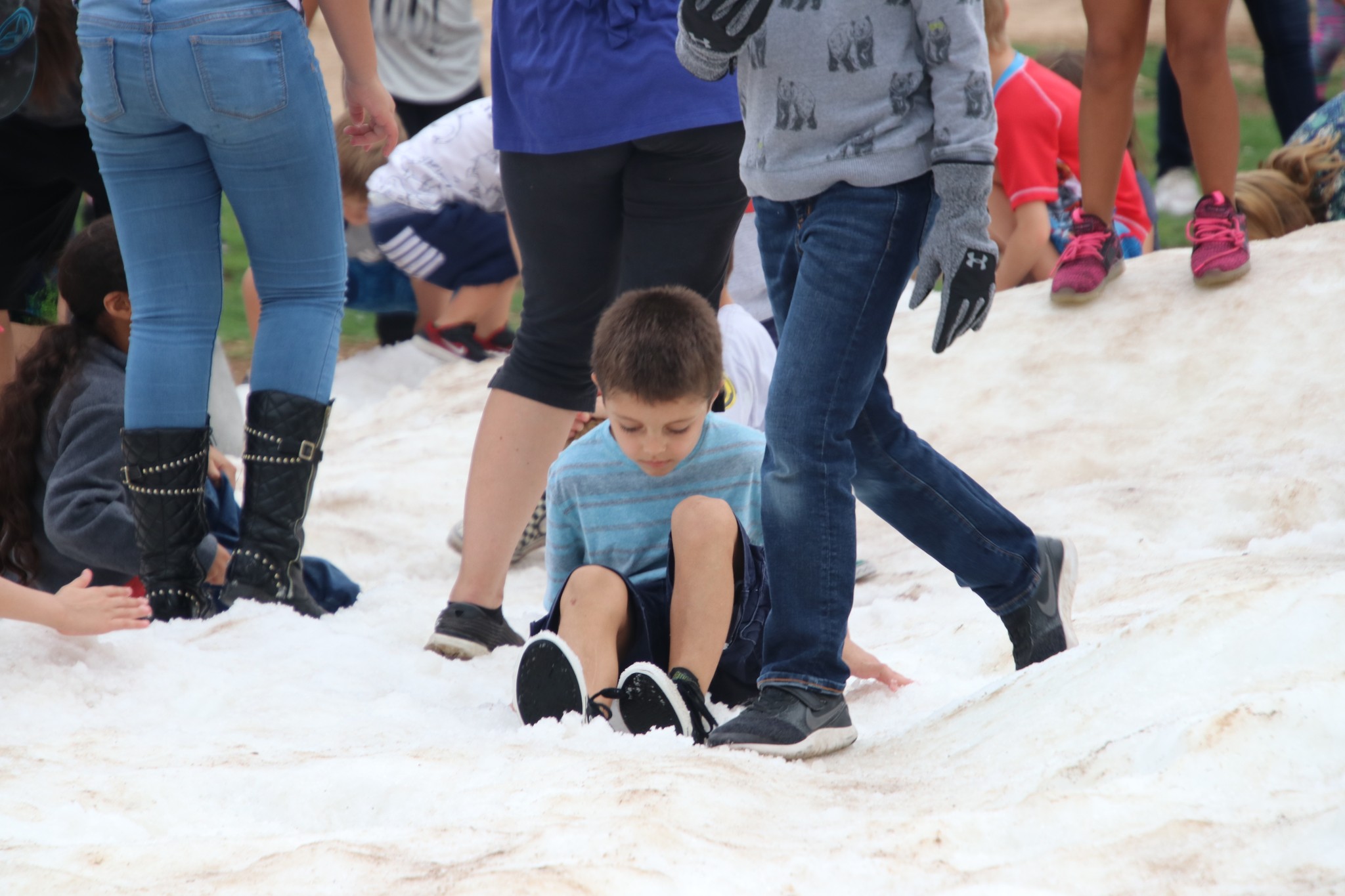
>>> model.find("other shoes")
[616,660,719,743]
[514,631,612,729]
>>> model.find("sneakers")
[1184,190,1253,285]
[415,320,497,367]
[475,322,517,356]
[707,683,857,760]
[998,535,1078,667]
[1047,209,1125,302]
[446,492,548,563]
[424,602,526,662]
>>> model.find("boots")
[219,391,330,618]
[120,413,228,619]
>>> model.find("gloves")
[678,0,772,61]
[908,159,999,354]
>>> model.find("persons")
[0,0,1345,759]
[515,284,912,744]
